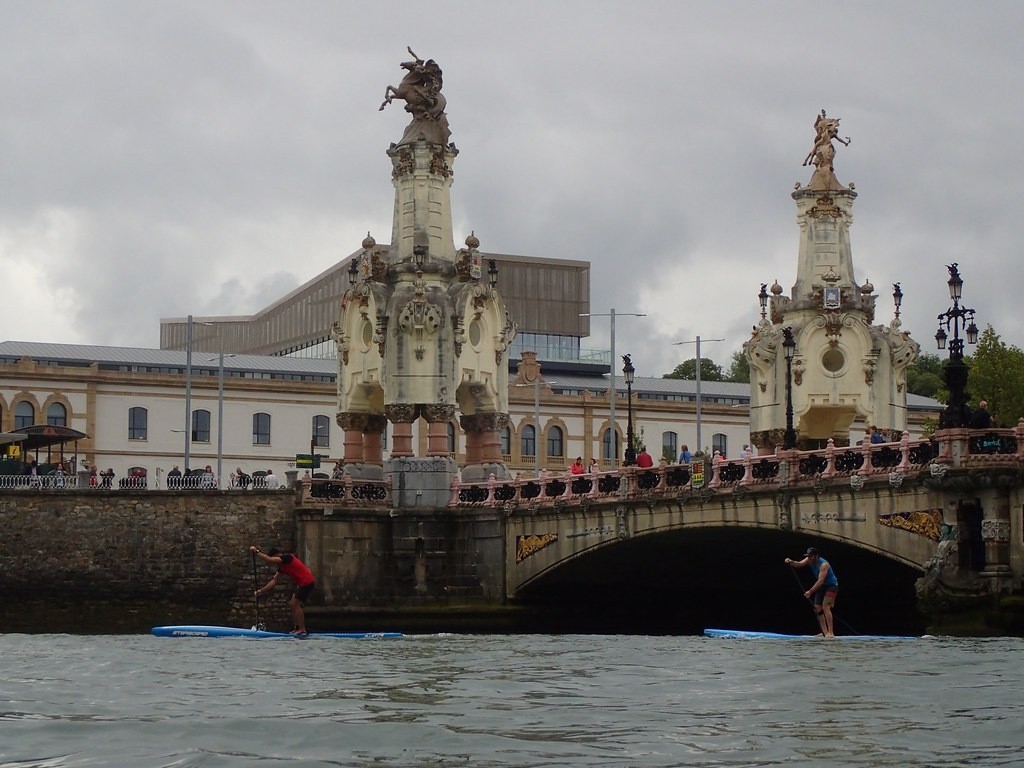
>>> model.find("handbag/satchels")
[681,451,687,464]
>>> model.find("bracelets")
[261,589,265,592]
[254,549,260,553]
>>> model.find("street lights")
[171,321,212,476]
[621,354,636,466]
[516,381,559,478]
[207,355,236,490]
[936,261,979,426]
[579,312,648,470]
[672,340,724,450]
[777,326,798,451]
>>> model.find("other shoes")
[289,629,307,636]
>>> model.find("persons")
[973,401,999,426]
[869,426,899,444]
[678,445,691,464]
[740,445,752,457]
[100,468,115,487]
[202,465,213,485]
[235,468,252,486]
[720,454,726,459]
[636,446,653,467]
[570,457,600,474]
[26,460,67,486]
[264,469,278,488]
[250,545,316,634]
[784,547,838,638]
[167,465,195,487]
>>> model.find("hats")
[268,548,279,556]
[803,547,819,556]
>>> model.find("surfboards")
[149,625,404,639]
[703,626,917,641]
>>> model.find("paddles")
[252,550,259,631]
[786,561,826,637]
